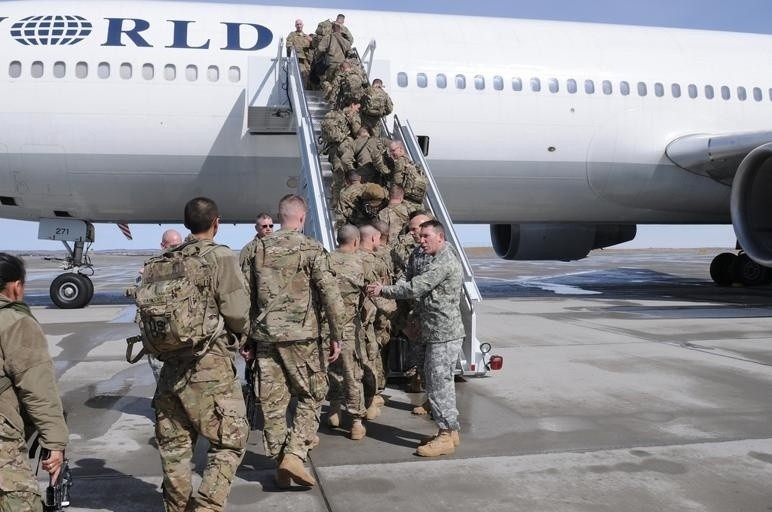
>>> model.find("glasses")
[262,224,274,228]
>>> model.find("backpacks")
[401,155,427,204]
[321,111,351,143]
[133,240,229,354]
[316,18,332,37]
[339,70,366,103]
[355,184,389,217]
[365,87,393,116]
[366,139,395,175]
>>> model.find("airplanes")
[1,0,771,313]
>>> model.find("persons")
[238,210,276,269]
[134,228,185,386]
[0,251,71,512]
[241,193,346,490]
[338,141,424,245]
[287,13,353,100]
[148,196,255,511]
[323,56,394,208]
[288,209,463,455]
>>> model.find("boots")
[351,419,366,440]
[275,452,316,486]
[329,398,343,426]
[406,374,420,392]
[413,399,431,414]
[417,426,460,456]
[365,393,385,418]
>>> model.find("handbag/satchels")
[314,52,330,75]
